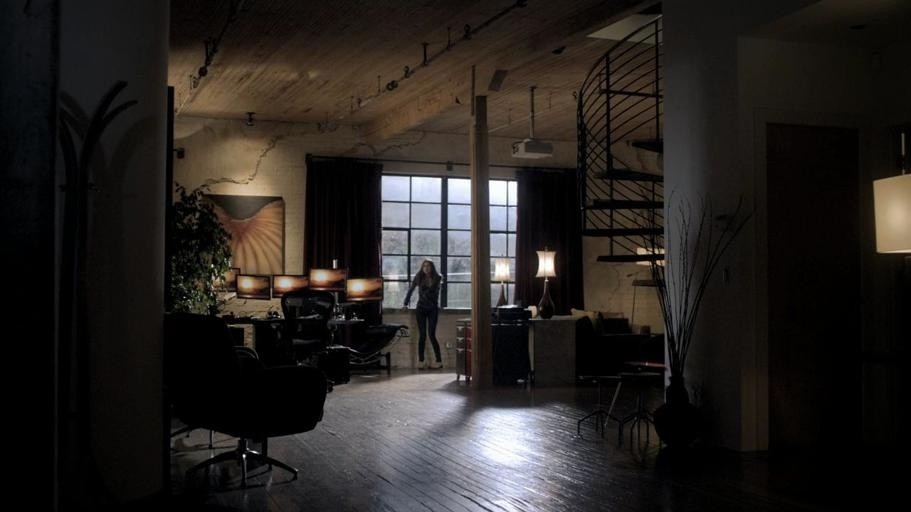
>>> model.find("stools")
[574,361,663,461]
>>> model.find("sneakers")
[430,362,444,369]
[417,361,426,369]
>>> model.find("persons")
[402,259,445,369]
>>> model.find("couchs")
[533,311,659,387]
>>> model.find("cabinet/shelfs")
[492,323,587,387]
[456,319,475,385]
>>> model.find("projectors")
[512,139,556,159]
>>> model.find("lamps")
[872,157,911,255]
[533,243,559,321]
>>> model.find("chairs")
[166,312,242,450]
[286,294,404,385]
[181,315,331,484]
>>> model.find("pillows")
[573,308,604,337]
